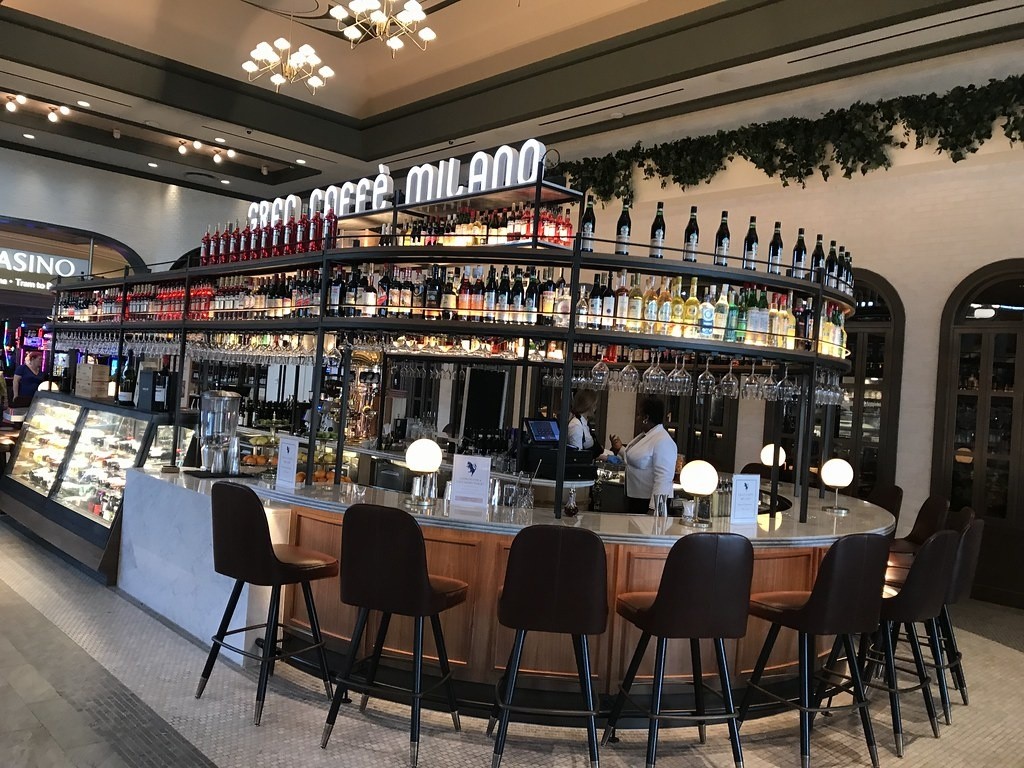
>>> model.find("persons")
[568,390,620,464]
[0,373,8,426]
[609,397,677,517]
[12,351,43,408]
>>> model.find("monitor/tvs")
[524,417,561,444]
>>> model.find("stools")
[194,463,985,768]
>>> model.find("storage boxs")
[76,363,110,382]
[75,380,108,398]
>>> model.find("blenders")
[199,389,242,471]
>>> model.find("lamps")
[329,0,436,59]
[241,10,335,96]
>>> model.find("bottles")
[326,262,572,329]
[564,482,579,517]
[208,368,267,385]
[714,210,730,266]
[57,267,322,323]
[238,395,295,427]
[742,216,855,297]
[649,202,666,259]
[575,268,816,353]
[683,207,700,263]
[462,427,518,476]
[615,198,632,256]
[411,410,438,443]
[818,300,848,359]
[580,194,596,252]
[199,199,338,266]
[378,201,573,247]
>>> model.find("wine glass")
[542,345,802,404]
[815,366,845,407]
[54,330,542,381]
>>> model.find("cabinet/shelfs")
[47,161,857,518]
[0,389,200,586]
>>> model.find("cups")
[444,480,452,516]
[503,472,535,508]
[211,451,225,474]
[653,494,670,519]
[488,477,501,513]
[225,437,241,475]
